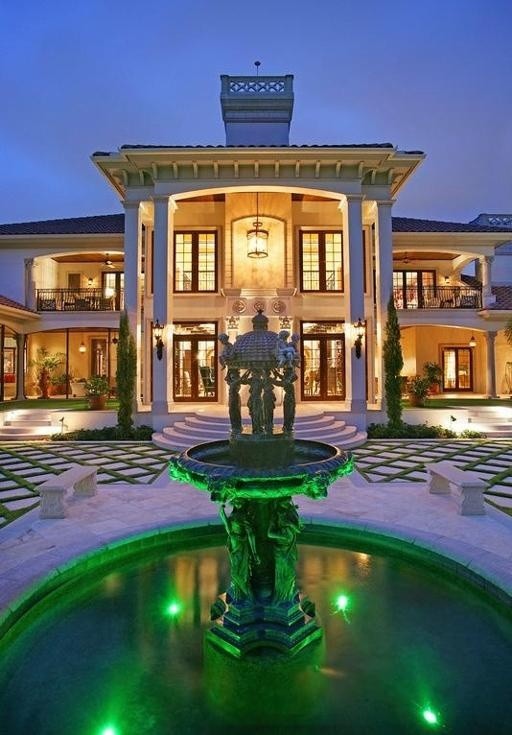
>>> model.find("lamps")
[469,332,477,348]
[78,333,86,355]
[152,318,165,342]
[353,317,368,338]
[245,192,269,258]
[87,277,93,286]
[445,276,450,284]
